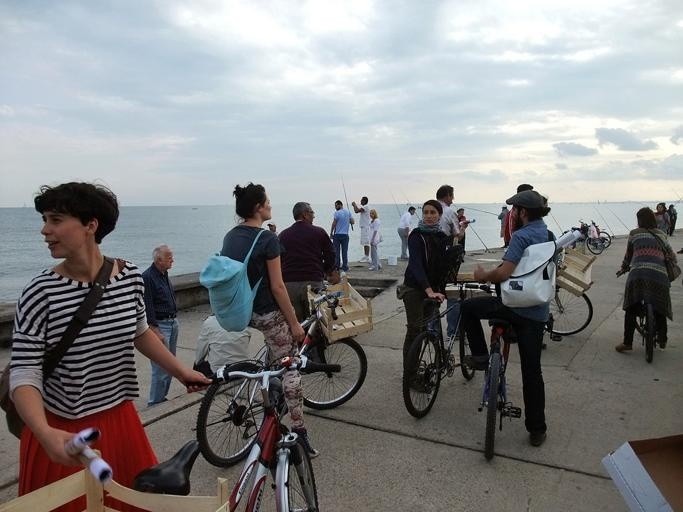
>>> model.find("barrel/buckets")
[387,255,397,265]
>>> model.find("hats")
[506,190,546,209]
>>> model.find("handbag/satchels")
[0,353,44,439]
[192,360,214,381]
[665,255,682,282]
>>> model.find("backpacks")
[500,241,557,307]
[198,228,265,332]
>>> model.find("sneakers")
[358,257,370,263]
[529,431,548,446]
[464,353,490,370]
[616,343,633,352]
[408,378,433,395]
[657,336,667,349]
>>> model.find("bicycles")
[473,317,530,465]
[559,219,612,257]
[543,280,593,353]
[397,279,492,421]
[614,266,661,363]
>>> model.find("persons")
[8,182,215,511]
[427,185,465,339]
[499,206,508,250]
[665,205,678,236]
[508,184,533,237]
[655,202,669,236]
[220,182,321,460]
[267,222,279,237]
[192,314,255,376]
[395,200,456,395]
[616,207,677,353]
[460,189,558,446]
[329,196,476,270]
[279,201,337,365]
[141,245,179,407]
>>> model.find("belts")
[155,314,177,319]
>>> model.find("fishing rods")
[452,203,489,252]
[342,175,354,231]
[594,207,615,238]
[460,206,498,216]
[549,212,563,235]
[403,192,421,222]
[388,187,401,219]
[607,208,631,232]
